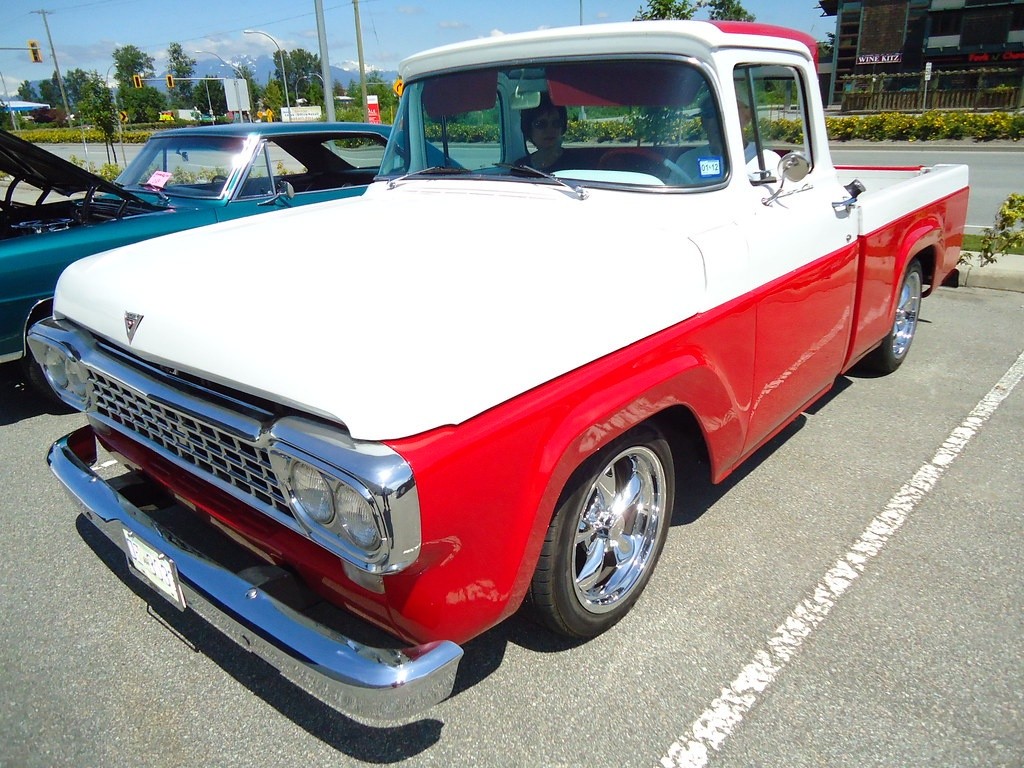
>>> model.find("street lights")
[195,51,245,123]
[220,63,245,80]
[243,30,292,123]
[295,76,307,107]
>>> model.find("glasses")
[530,119,567,130]
[699,108,720,120]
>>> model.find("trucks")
[156,108,214,123]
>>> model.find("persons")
[509,92,596,177]
[667,81,781,186]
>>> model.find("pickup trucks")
[18,20,970,728]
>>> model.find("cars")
[0,118,467,416]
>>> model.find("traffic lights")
[168,75,172,88]
[135,76,141,88]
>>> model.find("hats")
[686,81,753,122]
